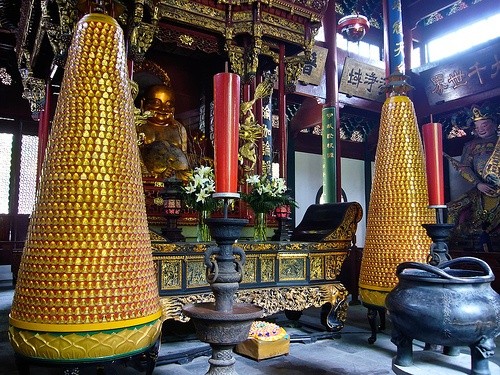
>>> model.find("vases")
[253,212,269,243]
[197,210,211,242]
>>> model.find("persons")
[443,105,500,236]
[136,85,196,178]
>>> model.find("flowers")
[183,167,235,240]
[240,175,299,240]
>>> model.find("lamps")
[160,175,184,242]
[274,202,295,242]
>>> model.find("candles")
[321,106,337,204]
[213,61,241,192]
[422,114,444,205]
